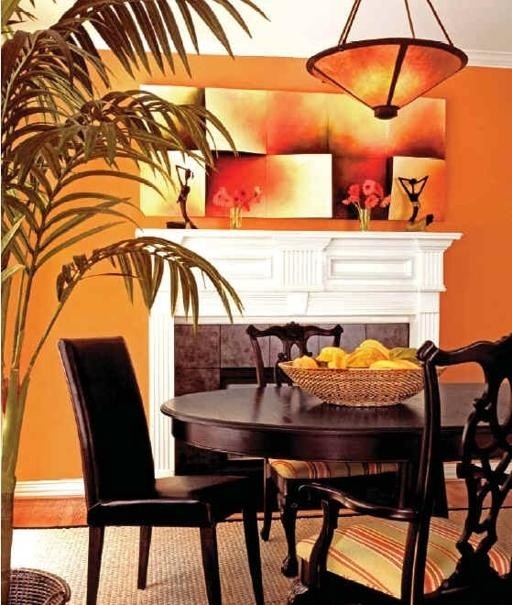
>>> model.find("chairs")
[58,337,265,605]
[246,321,410,579]
[287,333,511,605]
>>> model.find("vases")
[230,208,242,230]
[213,186,262,211]
[358,208,371,232]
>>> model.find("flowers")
[342,180,391,208]
[307,1,469,117]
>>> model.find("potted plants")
[3,1,272,605]
